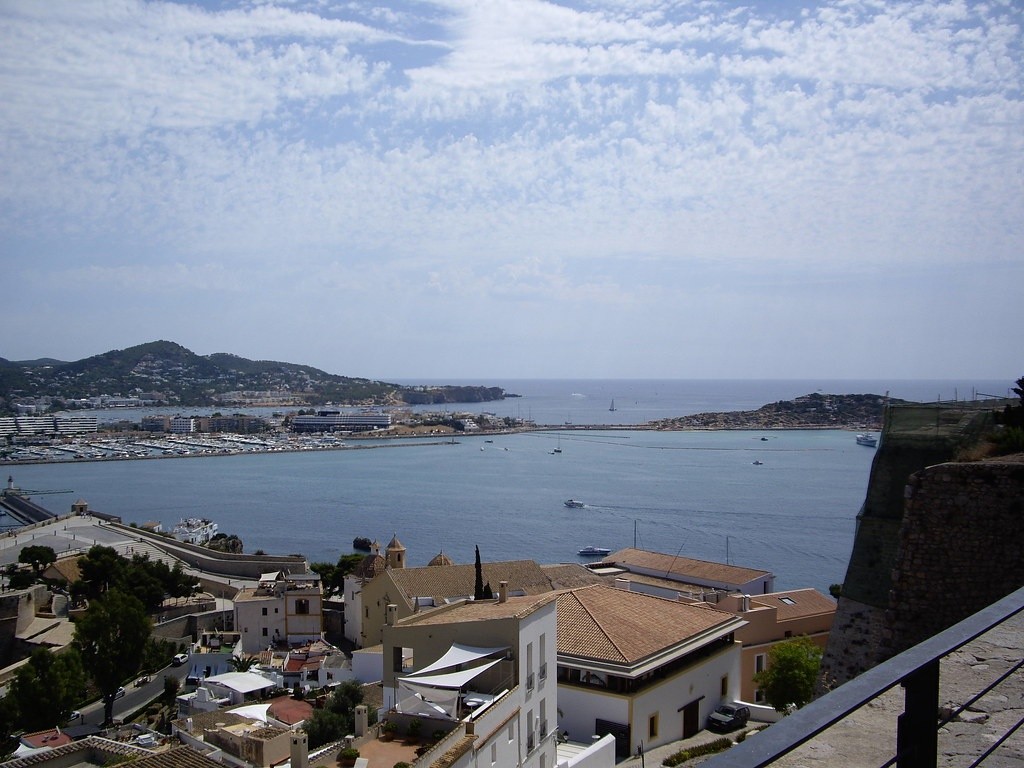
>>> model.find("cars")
[103,686,125,700]
[60,710,81,723]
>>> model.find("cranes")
[0,489,75,497]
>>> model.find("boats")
[484,440,493,443]
[856,432,878,447]
[761,438,768,441]
[553,448,562,452]
[753,461,763,465]
[576,546,615,555]
[564,499,584,508]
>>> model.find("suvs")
[708,702,751,733]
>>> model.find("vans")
[173,654,188,665]
